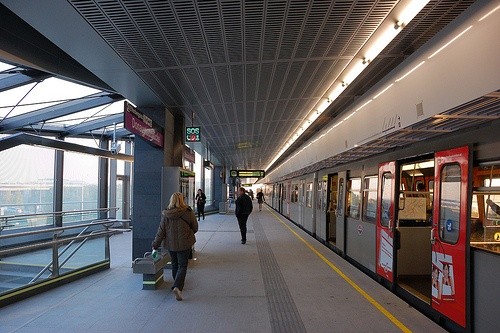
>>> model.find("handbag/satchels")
[199,200,206,205]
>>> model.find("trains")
[262,91,499,333]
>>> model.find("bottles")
[151,249,158,259]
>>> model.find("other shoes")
[241,238,246,244]
[198,218,200,221]
[203,218,204,220]
[260,210,261,211]
[173,288,182,301]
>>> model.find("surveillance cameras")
[111,144,121,155]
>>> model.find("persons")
[432,263,454,300]
[487,199,500,216]
[235,187,253,244]
[196,189,206,221]
[229,189,265,211]
[152,192,198,301]
[415,184,432,224]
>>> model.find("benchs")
[132,248,170,291]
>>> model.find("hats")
[239,188,245,191]
